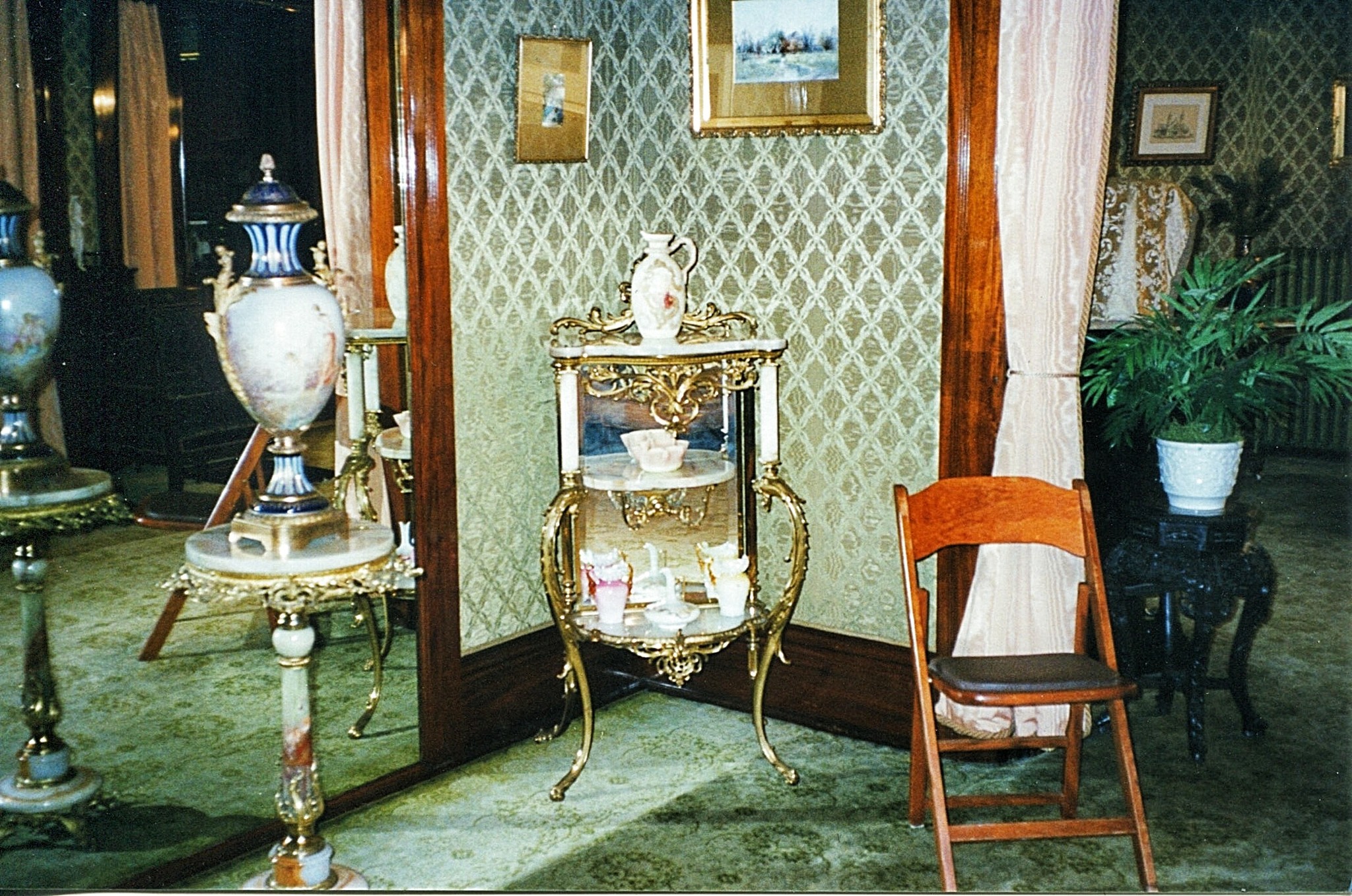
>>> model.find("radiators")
[1249,248,1352,471]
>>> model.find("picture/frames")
[1125,79,1221,165]
[512,33,592,165]
[689,0,887,139]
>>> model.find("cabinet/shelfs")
[325,327,416,735]
[540,233,810,804]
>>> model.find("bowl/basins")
[644,600,700,632]
[392,410,411,439]
[621,429,688,471]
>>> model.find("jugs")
[631,231,697,345]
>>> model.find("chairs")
[1077,176,1203,425]
[892,475,1159,895]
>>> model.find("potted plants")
[1079,254,1352,511]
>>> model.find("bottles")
[384,225,407,332]
[0,166,69,480]
[203,153,362,557]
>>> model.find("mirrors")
[0,0,469,895]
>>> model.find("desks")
[158,524,398,890]
[0,467,124,855]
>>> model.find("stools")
[1103,509,1278,763]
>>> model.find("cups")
[696,547,750,617]
[584,551,631,626]
